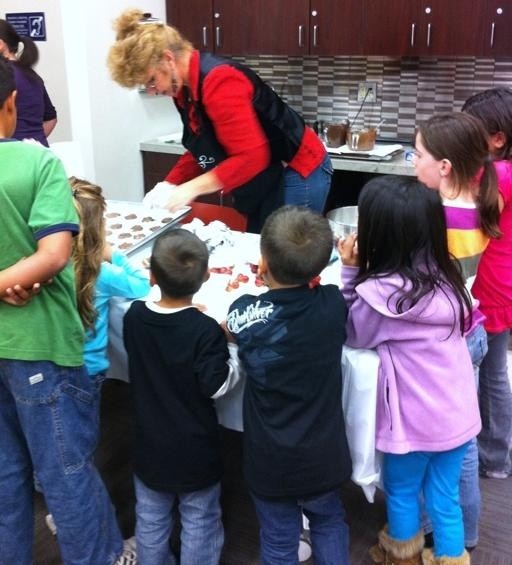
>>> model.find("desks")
[104,198,480,505]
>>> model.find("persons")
[104,7,333,232]
[336,173,485,564]
[1,49,143,565]
[121,227,243,564]
[407,110,502,551]
[64,172,153,470]
[459,84,512,483]
[0,19,60,151]
[218,202,348,565]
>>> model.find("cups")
[323,120,377,151]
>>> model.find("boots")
[368,523,424,565]
[424,547,468,565]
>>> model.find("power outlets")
[359,83,377,104]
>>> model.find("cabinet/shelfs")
[483,0,512,60]
[364,1,485,58]
[262,1,363,56]
[165,0,262,56]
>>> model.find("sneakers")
[115,530,138,563]
[477,465,509,479]
